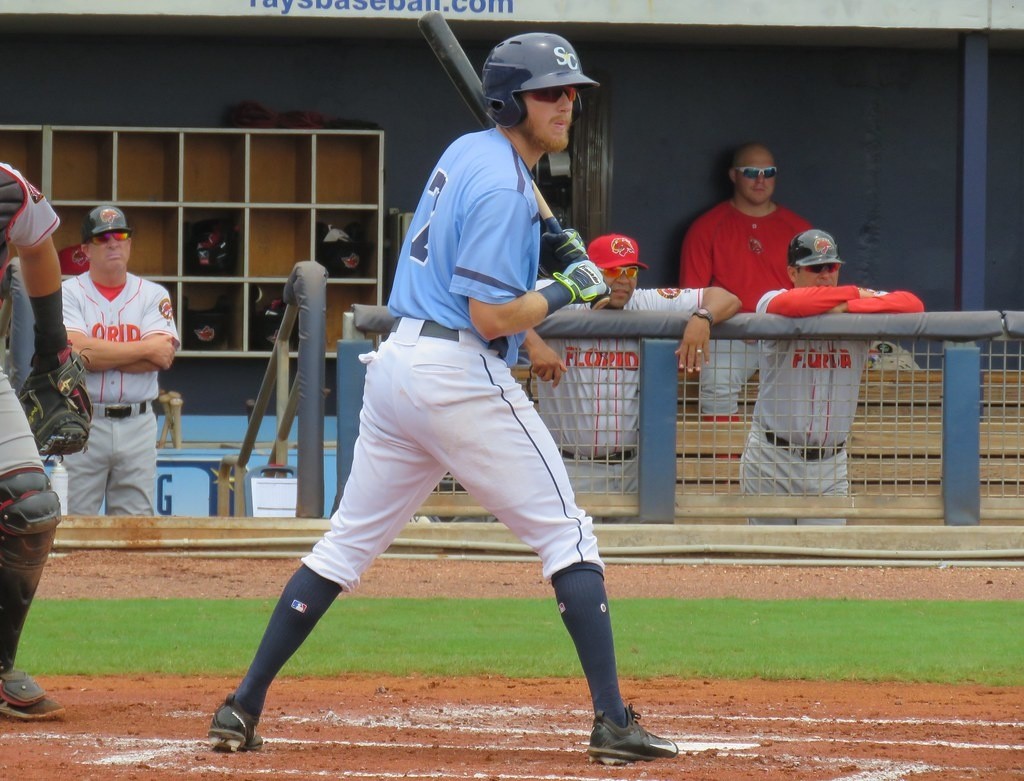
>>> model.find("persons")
[0,161,92,721]
[740,229,923,526]
[677,144,814,458]
[207,33,679,765]
[523,233,741,524]
[61,205,180,516]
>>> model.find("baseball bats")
[418,10,611,311]
[157,389,184,449]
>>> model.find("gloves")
[552,260,612,305]
[540,225,590,278]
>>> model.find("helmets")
[81,205,131,243]
[786,229,844,267]
[482,32,600,127]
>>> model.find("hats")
[587,233,648,269]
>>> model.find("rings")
[696,350,702,352]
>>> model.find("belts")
[390,318,487,348]
[764,433,845,460]
[561,449,636,465]
[88,403,146,418]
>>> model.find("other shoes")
[0,670,65,721]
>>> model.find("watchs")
[692,308,713,326]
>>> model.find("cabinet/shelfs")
[0,125,385,360]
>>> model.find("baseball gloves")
[21,352,93,455]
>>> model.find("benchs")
[404,364,1024,520]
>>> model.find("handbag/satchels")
[249,287,296,351]
[182,296,234,350]
[57,244,90,275]
[315,221,365,277]
[185,213,236,276]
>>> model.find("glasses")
[795,262,841,273]
[89,230,132,245]
[597,266,639,280]
[734,165,777,179]
[522,85,577,104]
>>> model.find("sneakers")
[208,692,263,753]
[586,703,679,766]
